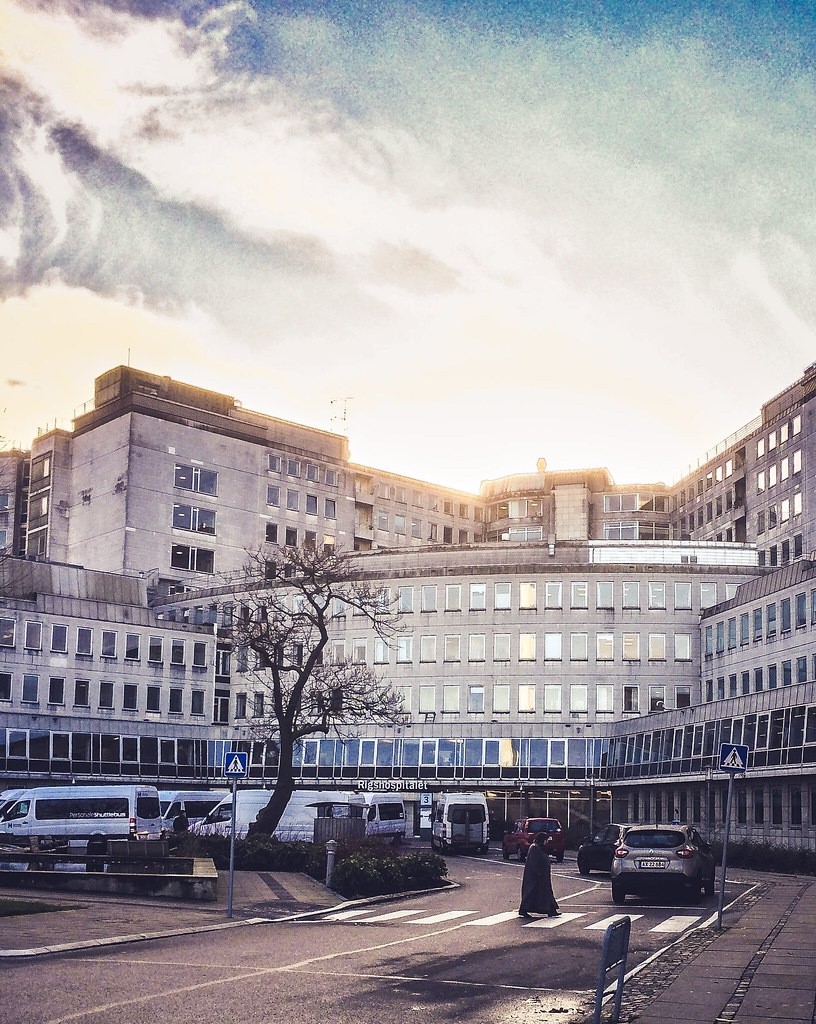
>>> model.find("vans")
[189,789,365,848]
[330,792,406,841]
[428,792,490,855]
[0,785,161,849]
[159,789,232,835]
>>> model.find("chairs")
[44,834,68,854]
[29,836,56,853]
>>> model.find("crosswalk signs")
[718,743,750,774]
[224,752,248,778]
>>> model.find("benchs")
[106,839,177,857]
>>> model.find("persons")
[519,832,562,919]
[172,810,189,833]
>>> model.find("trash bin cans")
[306,801,375,843]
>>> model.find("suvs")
[503,817,566,862]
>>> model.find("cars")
[611,826,716,905]
[577,824,644,876]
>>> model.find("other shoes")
[548,910,562,917]
[519,912,532,918]
